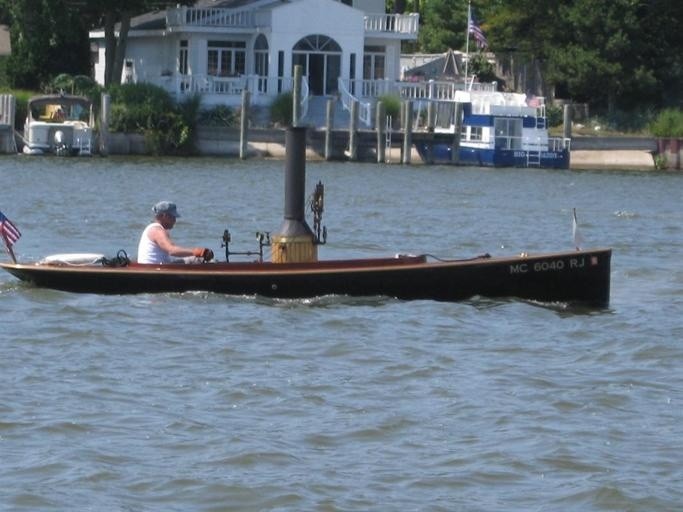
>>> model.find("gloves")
[202,247,214,261]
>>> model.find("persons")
[135,201,215,265]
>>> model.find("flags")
[0,211,22,252]
[466,4,489,51]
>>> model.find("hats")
[150,200,181,217]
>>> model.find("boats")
[412,74,573,172]
[0,246,616,310]
[20,89,96,155]
